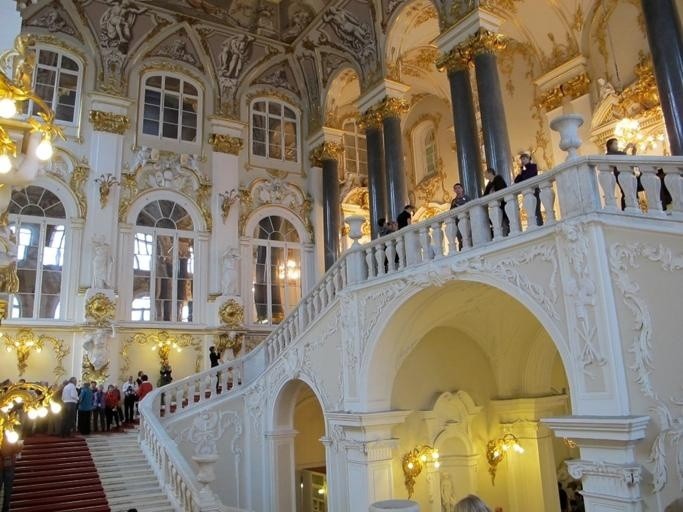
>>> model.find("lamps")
[0,326,63,451]
[0,33,68,174]
[486,433,524,487]
[402,444,440,501]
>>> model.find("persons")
[1,369,153,435]
[209,346,220,389]
[448,183,472,250]
[557,481,584,511]
[514,153,543,225]
[483,168,510,232]
[374,217,394,271]
[397,205,413,230]
[605,138,640,210]
